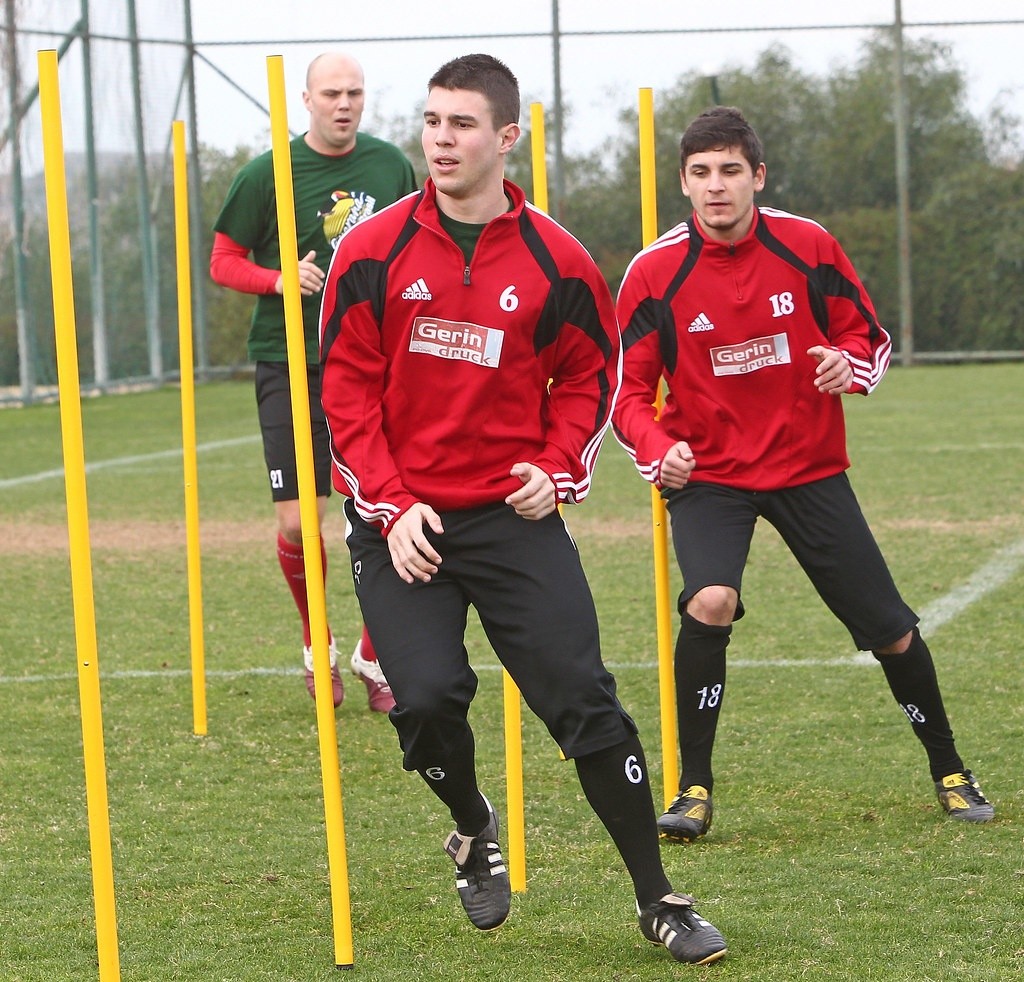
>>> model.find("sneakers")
[442,789,512,931]
[302,625,345,710]
[349,638,395,714]
[634,892,728,965]
[934,769,996,823]
[656,784,713,841]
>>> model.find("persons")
[611,108,994,844]
[209,50,417,712]
[318,54,726,966]
